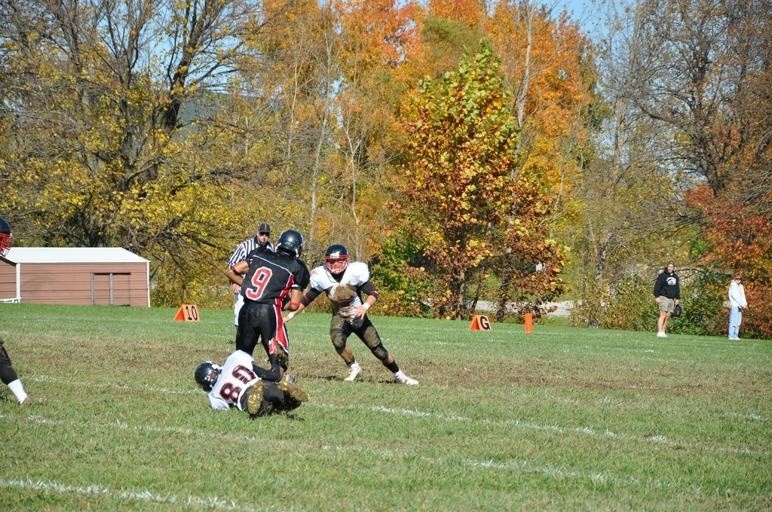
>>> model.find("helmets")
[0,217,12,257]
[194,362,222,393]
[278,229,303,257]
[324,243,349,275]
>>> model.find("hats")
[258,222,271,235]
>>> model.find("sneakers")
[657,331,667,337]
[395,374,419,386]
[246,382,265,416]
[343,363,361,380]
[278,381,310,404]
[729,335,742,342]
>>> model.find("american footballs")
[282,293,291,311]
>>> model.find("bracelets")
[287,313,294,318]
[361,302,370,310]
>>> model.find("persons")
[194,349,310,418]
[727,272,749,341]
[227,222,275,347]
[224,229,310,384]
[279,243,420,386]
[653,259,681,337]
[0,337,28,404]
